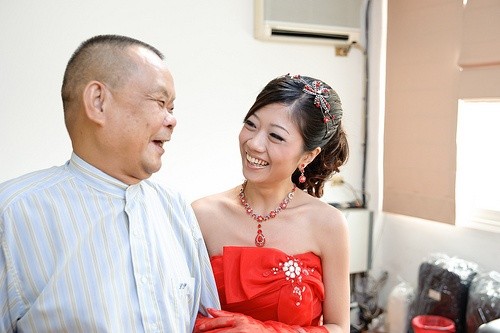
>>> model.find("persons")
[190,72,351,333]
[1,34,222,333]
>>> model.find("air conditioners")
[254,0,361,46]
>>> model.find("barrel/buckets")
[412,314,456,333]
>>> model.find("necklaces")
[238,179,297,248]
